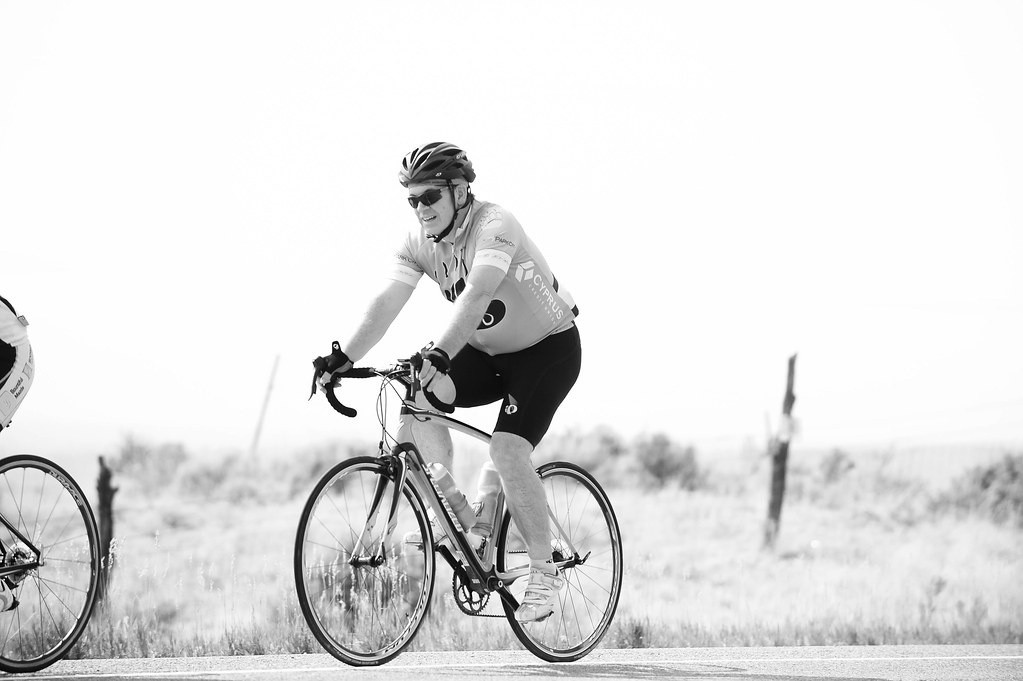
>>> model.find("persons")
[317,140,582,623]
[0,294,36,613]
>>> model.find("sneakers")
[405,495,475,545]
[515,567,563,621]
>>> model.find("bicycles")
[294,339,626,665]
[0,420,105,675]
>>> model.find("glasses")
[407,186,451,208]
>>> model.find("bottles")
[427,461,478,532]
[470,458,501,536]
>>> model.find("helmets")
[399,142,475,186]
